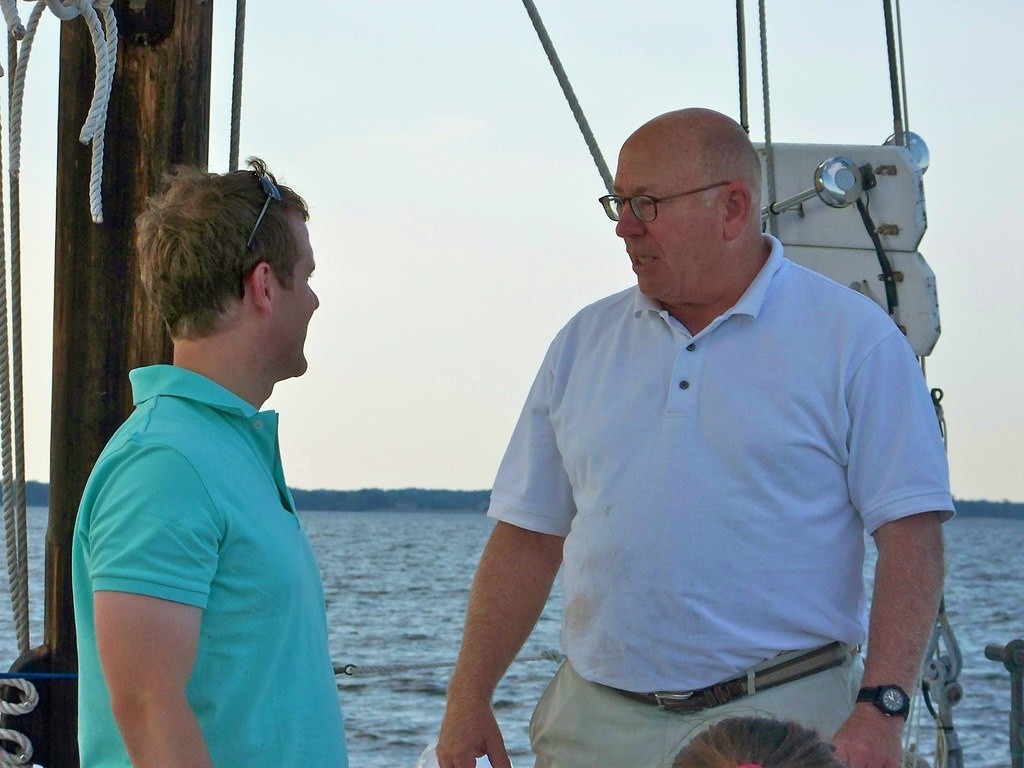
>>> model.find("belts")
[614,639,860,714]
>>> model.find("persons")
[671,715,847,768]
[435,108,956,768]
[71,156,373,768]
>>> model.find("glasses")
[598,180,730,222]
[234,170,282,300]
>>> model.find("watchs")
[856,685,911,722]
